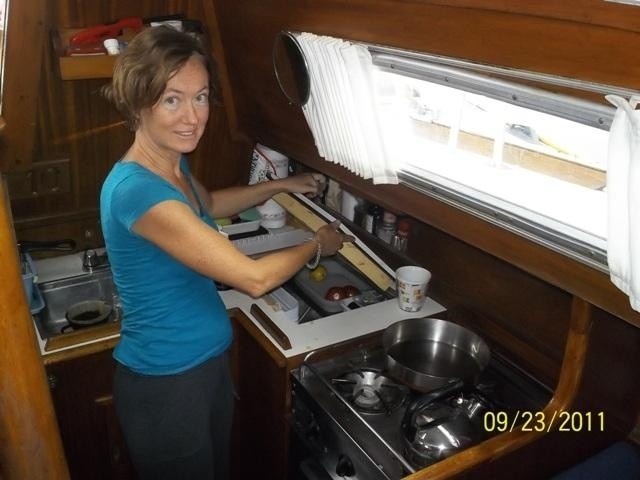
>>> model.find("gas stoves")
[288,345,556,480]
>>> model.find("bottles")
[392,220,410,254]
[354,197,367,228]
[365,203,382,235]
[103,38,120,55]
[377,211,397,244]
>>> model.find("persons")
[99,24,355,479]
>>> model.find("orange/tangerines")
[309,265,329,283]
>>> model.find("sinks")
[32,268,124,340]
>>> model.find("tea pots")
[401,376,497,467]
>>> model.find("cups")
[396,266,433,313]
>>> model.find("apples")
[326,286,346,302]
[345,285,361,298]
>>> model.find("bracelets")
[302,236,322,270]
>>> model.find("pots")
[383,318,525,428]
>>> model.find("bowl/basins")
[66,300,112,330]
